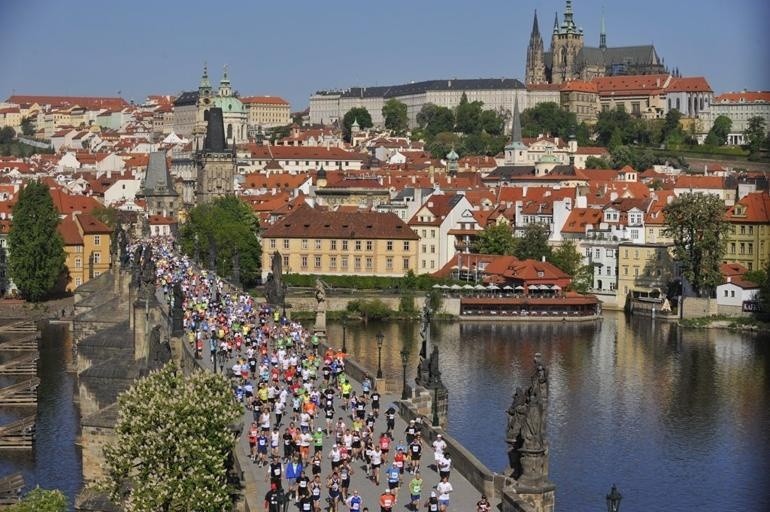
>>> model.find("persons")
[503,351,553,453]
[126,234,495,512]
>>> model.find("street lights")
[282,281,287,317]
[606,483,623,512]
[341,312,347,354]
[376,328,384,378]
[265,477,290,512]
[400,347,410,399]
[157,274,173,318]
[193,320,218,373]
